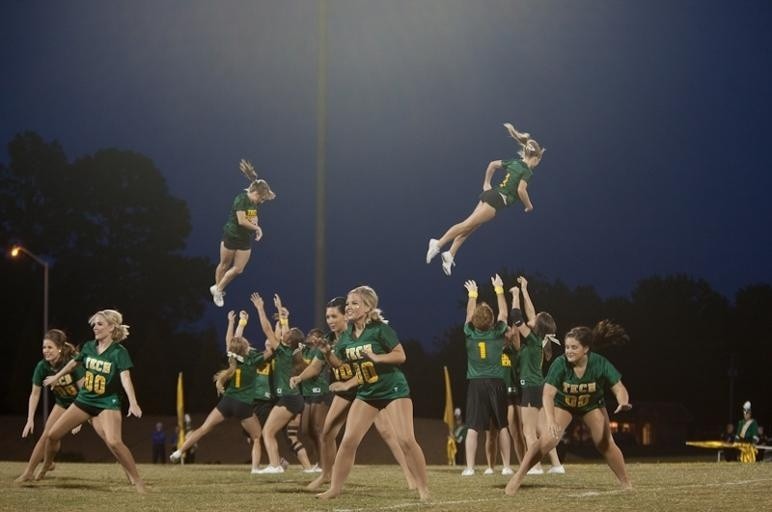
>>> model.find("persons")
[735,401,759,463]
[426,123,547,277]
[21,328,135,486]
[151,422,167,464]
[32,308,151,496]
[757,426,768,461]
[209,160,277,308]
[721,423,735,462]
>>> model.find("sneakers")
[302,462,322,473]
[425,238,441,265]
[460,464,567,477]
[250,457,288,474]
[209,285,227,308]
[170,448,182,461]
[440,250,456,277]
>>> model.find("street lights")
[10,244,50,440]
